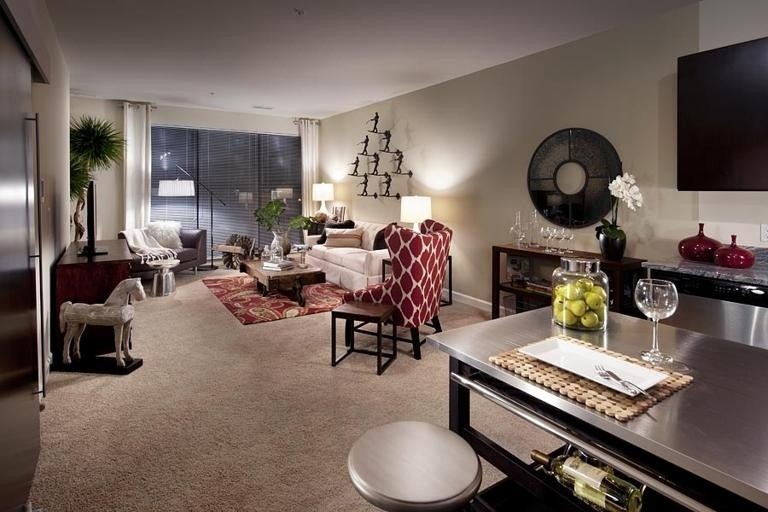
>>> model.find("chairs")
[341,220,453,359]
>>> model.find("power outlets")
[760,225,768,242]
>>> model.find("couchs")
[304,218,389,291]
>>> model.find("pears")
[554,278,607,327]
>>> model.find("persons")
[348,111,404,197]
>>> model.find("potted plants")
[252,200,312,256]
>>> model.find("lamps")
[238,191,253,210]
[313,182,334,216]
[277,187,292,211]
[157,164,226,271]
[400,196,432,233]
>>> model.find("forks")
[594,365,637,394]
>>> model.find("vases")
[677,223,722,261]
[600,230,627,257]
[714,235,754,269]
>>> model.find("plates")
[518,339,670,396]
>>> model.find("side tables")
[382,256,453,308]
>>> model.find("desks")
[56,239,132,357]
[640,245,768,351]
[492,243,648,319]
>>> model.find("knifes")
[606,370,647,395]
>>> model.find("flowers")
[597,169,644,228]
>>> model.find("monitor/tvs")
[77,181,109,256]
[675,34,767,192]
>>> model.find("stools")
[346,419,483,511]
[330,302,397,375]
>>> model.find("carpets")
[201,276,348,325]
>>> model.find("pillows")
[148,221,183,249]
[317,220,397,250]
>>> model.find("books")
[263,266,294,271]
[264,261,294,268]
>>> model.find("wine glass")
[537,227,552,248]
[634,279,679,363]
[562,227,575,253]
[551,227,564,254]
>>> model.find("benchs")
[117,229,207,280]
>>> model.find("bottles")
[272,241,283,261]
[508,212,526,248]
[528,210,540,248]
[552,258,610,332]
[530,449,643,512]
[261,245,272,265]
